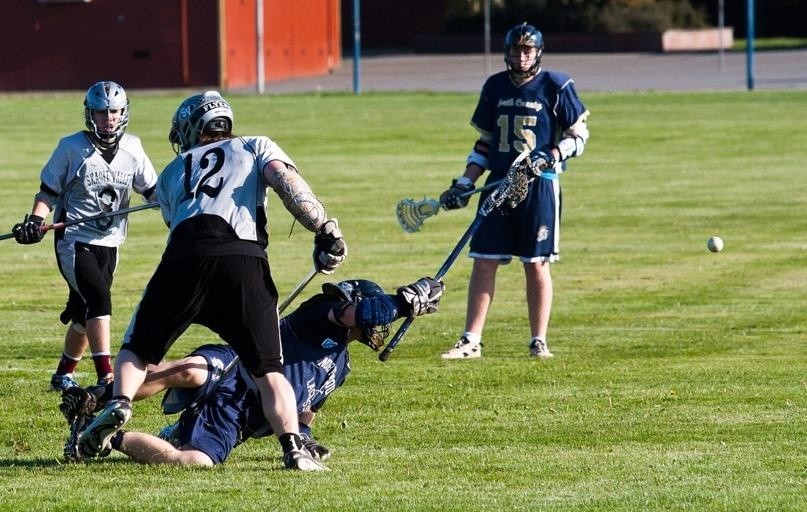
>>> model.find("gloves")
[440,177,474,210]
[397,277,446,317]
[12,214,46,243]
[313,217,347,274]
[520,147,555,177]
[300,433,329,459]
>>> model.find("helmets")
[323,279,391,352]
[83,81,130,148]
[503,22,544,78]
[169,91,233,156]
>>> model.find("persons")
[78,91,349,470]
[436,23,590,361]
[59,276,445,466]
[12,82,159,393]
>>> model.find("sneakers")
[284,450,331,472]
[442,336,480,359]
[59,387,97,425]
[528,337,553,358]
[64,415,94,462]
[75,399,132,460]
[50,373,79,392]
